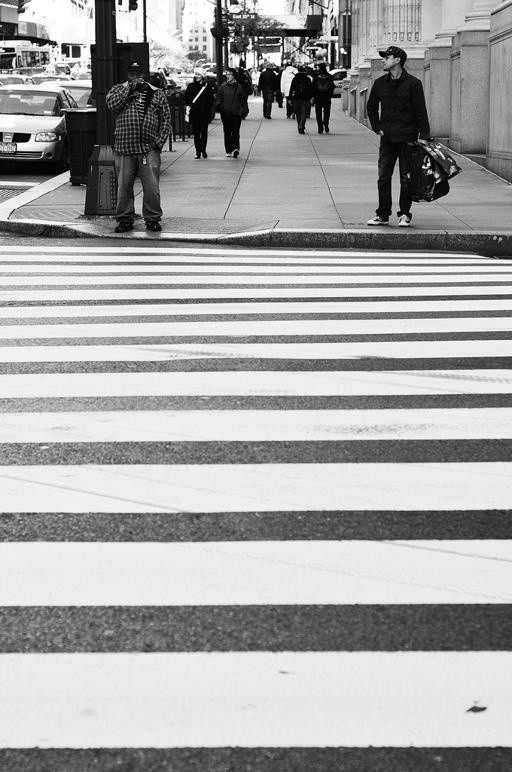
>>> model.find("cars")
[329,68,348,97]
[151,58,217,93]
[0,68,92,173]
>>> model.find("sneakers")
[225,153,231,158]
[202,151,207,159]
[396,214,411,228]
[365,214,391,227]
[194,154,201,159]
[232,149,240,158]
[144,222,162,232]
[113,222,134,234]
[317,121,323,134]
[298,127,305,135]
[263,114,311,120]
[324,122,330,133]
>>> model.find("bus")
[0,40,56,76]
[60,42,90,68]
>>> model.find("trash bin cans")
[59,108,99,186]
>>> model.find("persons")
[215,68,247,157]
[17,56,22,68]
[6,59,13,69]
[106,62,172,232]
[236,58,336,133]
[367,46,430,228]
[182,67,214,158]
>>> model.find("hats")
[194,67,206,78]
[378,46,408,64]
[127,61,146,70]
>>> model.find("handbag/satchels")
[183,106,192,124]
[404,139,451,205]
[414,135,464,181]
